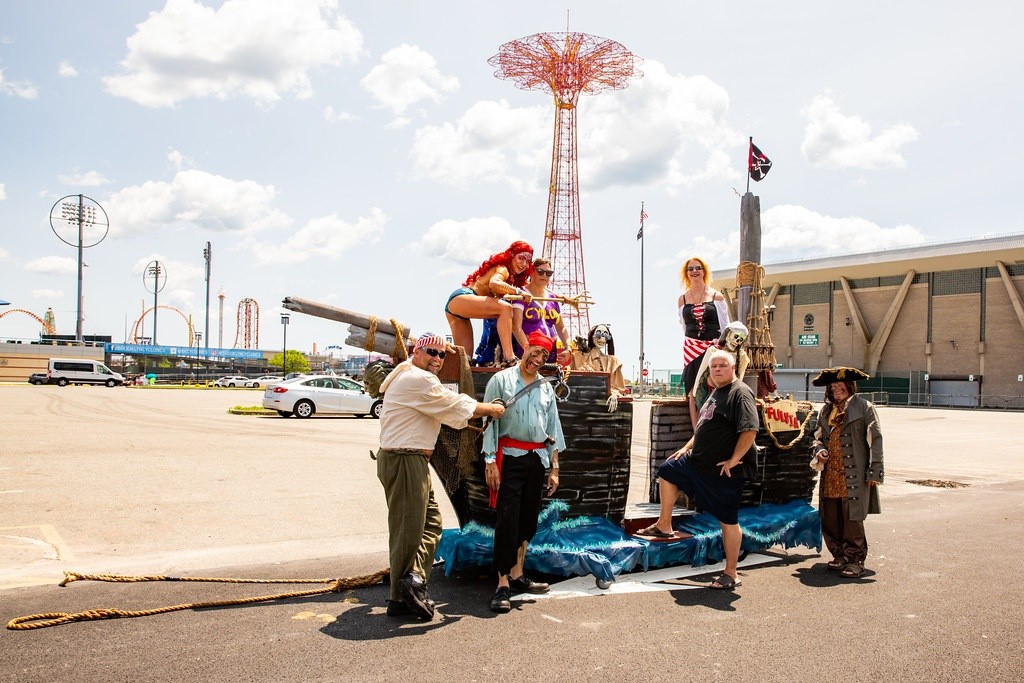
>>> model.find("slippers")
[709,573,743,589]
[636,524,675,537]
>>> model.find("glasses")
[687,266,703,271]
[421,347,446,359]
[536,268,554,277]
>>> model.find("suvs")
[222,376,248,387]
[28,373,57,385]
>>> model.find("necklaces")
[691,286,706,304]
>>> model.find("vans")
[47,358,122,388]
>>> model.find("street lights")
[281,313,289,378]
[195,332,202,384]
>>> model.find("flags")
[637,227,642,241]
[640,210,648,223]
[749,143,773,182]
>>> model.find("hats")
[811,365,871,387]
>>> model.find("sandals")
[828,557,866,578]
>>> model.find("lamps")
[949,340,956,348]
[845,318,852,326]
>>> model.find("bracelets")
[565,345,572,349]
[550,461,560,469]
[515,287,520,294]
[565,349,571,355]
[484,455,496,460]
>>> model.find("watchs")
[485,458,496,464]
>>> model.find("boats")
[283,134,819,580]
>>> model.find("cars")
[208,376,222,387]
[245,375,281,389]
[263,375,383,418]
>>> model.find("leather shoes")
[490,586,511,612]
[387,573,435,620]
[507,575,550,594]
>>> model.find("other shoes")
[501,356,519,367]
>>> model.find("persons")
[678,258,730,433]
[444,241,572,369]
[803,366,884,578]
[481,328,566,610]
[636,350,760,591]
[376,332,505,618]
